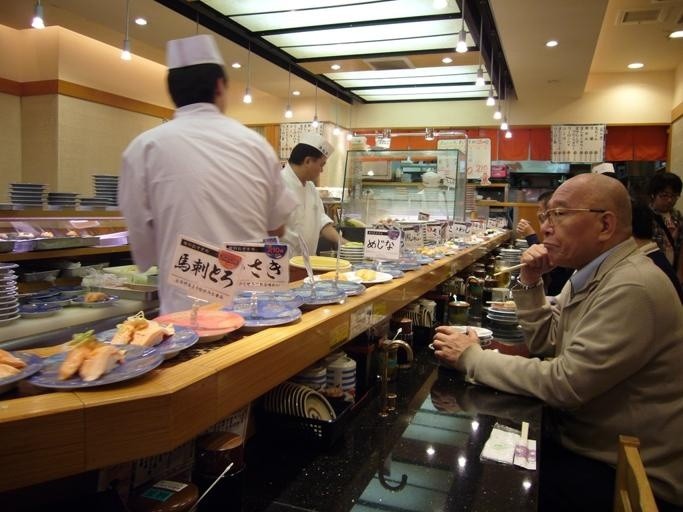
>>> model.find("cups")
[293,350,356,391]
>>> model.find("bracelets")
[515,275,543,291]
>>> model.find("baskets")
[255,369,382,451]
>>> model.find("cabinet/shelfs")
[0,209,132,293]
[362,181,509,213]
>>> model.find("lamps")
[122,0,132,59]
[31,0,44,29]
[285,65,292,118]
[347,105,352,139]
[312,81,319,127]
[353,128,464,140]
[244,40,252,103]
[455,0,512,138]
[334,90,339,134]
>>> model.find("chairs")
[613,434,658,512]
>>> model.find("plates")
[397,299,437,328]
[219,263,400,334]
[79,196,115,206]
[258,382,337,437]
[0,261,247,392]
[47,191,80,207]
[339,241,461,277]
[428,238,529,351]
[8,181,48,206]
[90,173,120,196]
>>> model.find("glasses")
[658,190,678,203]
[536,207,607,227]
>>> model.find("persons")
[118,34,299,313]
[647,171,682,285]
[431,172,682,511]
[280,131,349,264]
[516,191,575,296]
[632,202,683,306]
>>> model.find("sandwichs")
[111,315,174,347]
[58,339,128,382]
[85,293,109,303]
[0,348,26,378]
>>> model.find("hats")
[591,163,615,175]
[166,33,224,71]
[302,132,334,158]
